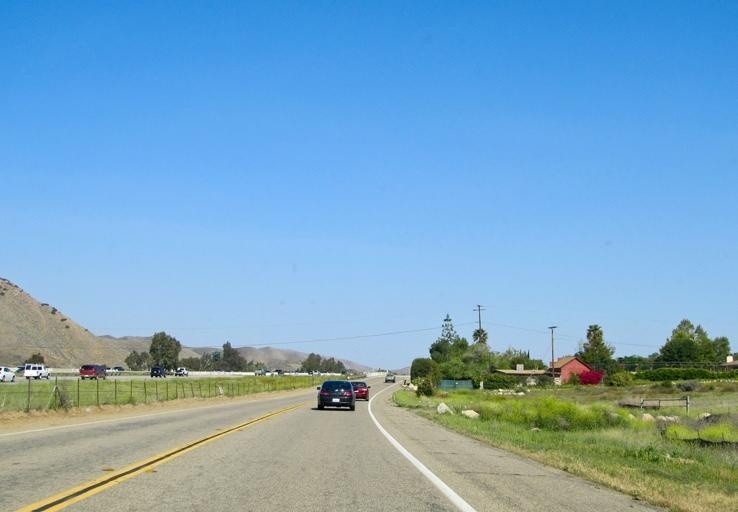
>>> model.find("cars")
[352,381,371,402]
[316,381,359,412]
[254,368,267,376]
[150,366,166,377]
[79,364,124,380]
[384,373,396,383]
[0,367,17,383]
[174,367,189,376]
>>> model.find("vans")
[24,363,52,379]
